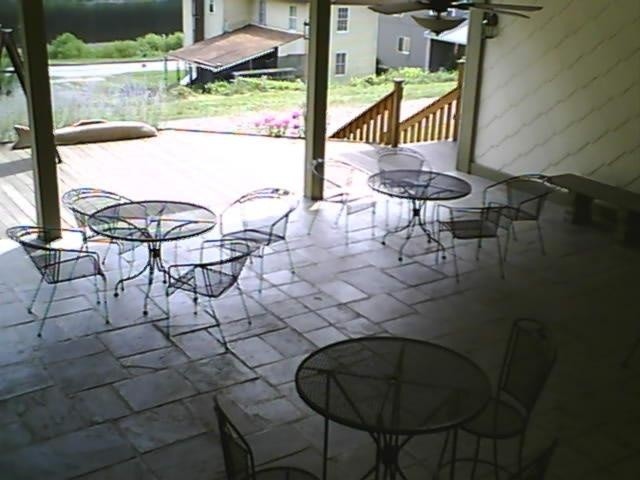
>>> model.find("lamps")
[481,11,498,39]
[409,11,468,38]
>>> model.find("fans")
[367,1,544,37]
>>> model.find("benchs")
[548,172,639,247]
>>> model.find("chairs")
[310,149,558,283]
[505,437,564,480]
[62,188,151,291]
[218,186,300,291]
[152,237,260,354]
[434,316,560,480]
[213,396,323,480]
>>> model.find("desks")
[6,224,113,338]
[88,200,218,316]
[295,336,490,480]
